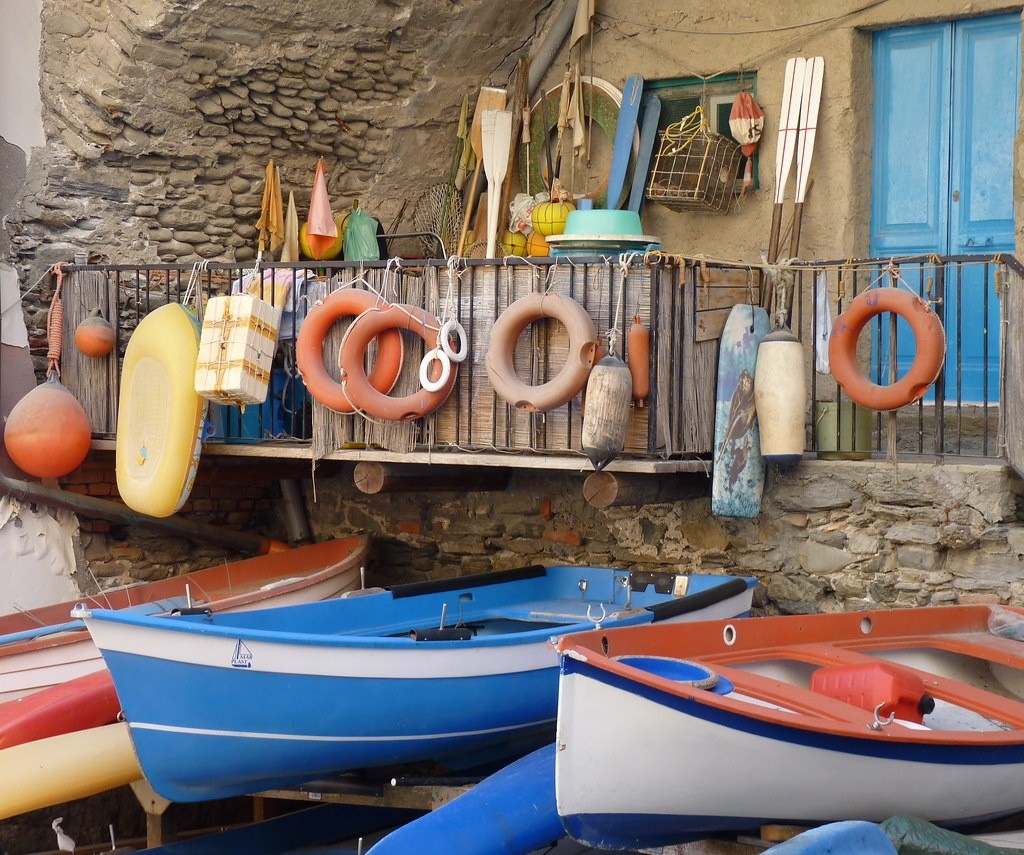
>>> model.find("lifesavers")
[295,288,404,415]
[484,291,598,415]
[828,287,948,412]
[339,303,459,422]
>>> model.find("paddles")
[762,57,807,320]
[607,73,644,211]
[628,94,662,211]
[455,86,507,258]
[480,109,513,259]
[781,56,825,333]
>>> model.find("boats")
[70,565,758,804]
[547,604,1023,851]
[0,533,371,820]
